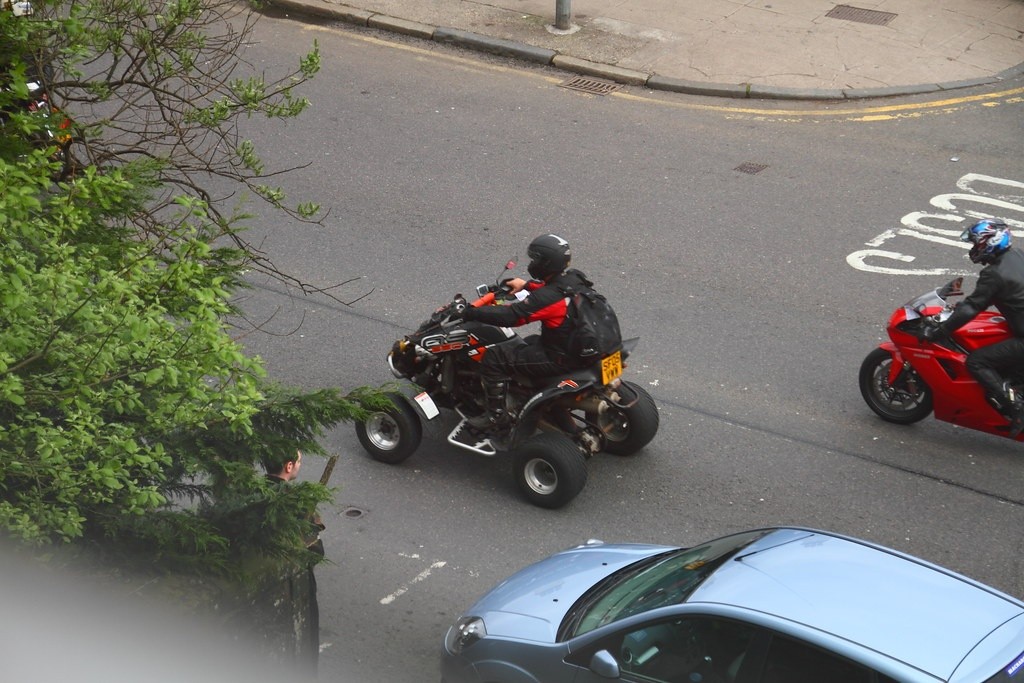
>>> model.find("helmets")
[527,233,571,279]
[959,219,1012,265]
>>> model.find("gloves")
[449,302,475,320]
[917,323,940,341]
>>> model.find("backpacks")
[545,269,622,368]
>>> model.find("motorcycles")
[354,256,661,509]
[860,277,1023,444]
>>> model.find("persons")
[259,442,326,683]
[0,25,53,99]
[457,233,591,429]
[930,219,1024,429]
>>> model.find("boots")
[468,378,512,433]
[986,380,1024,438]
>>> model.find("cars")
[438,523,1024,683]
